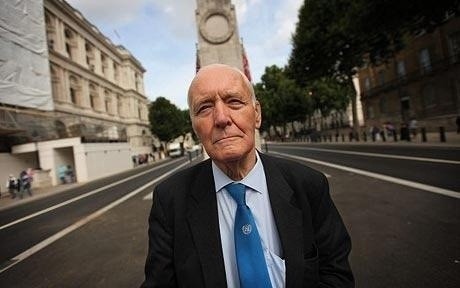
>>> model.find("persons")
[141,65,354,288]
[6,172,35,196]
[369,118,421,138]
[131,153,155,168]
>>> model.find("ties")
[224,183,275,287]
